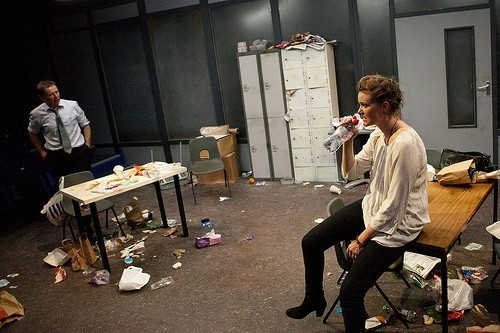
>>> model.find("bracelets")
[356,236,364,248]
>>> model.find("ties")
[49,107,72,154]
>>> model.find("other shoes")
[86,228,97,245]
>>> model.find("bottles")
[324,116,358,153]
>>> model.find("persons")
[28,80,91,229]
[286,74,431,333]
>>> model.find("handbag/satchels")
[40,176,70,226]
[435,159,476,185]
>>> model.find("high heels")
[286,289,327,319]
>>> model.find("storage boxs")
[198,134,238,184]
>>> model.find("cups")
[382,305,417,323]
[113,165,124,175]
[329,185,342,194]
[142,212,152,221]
[409,274,428,289]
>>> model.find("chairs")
[189,137,230,205]
[60,171,125,243]
[323,198,411,329]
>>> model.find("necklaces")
[386,118,398,145]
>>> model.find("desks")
[60,161,189,274]
[327,127,374,188]
[407,181,498,333]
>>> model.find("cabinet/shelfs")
[237,43,343,182]
